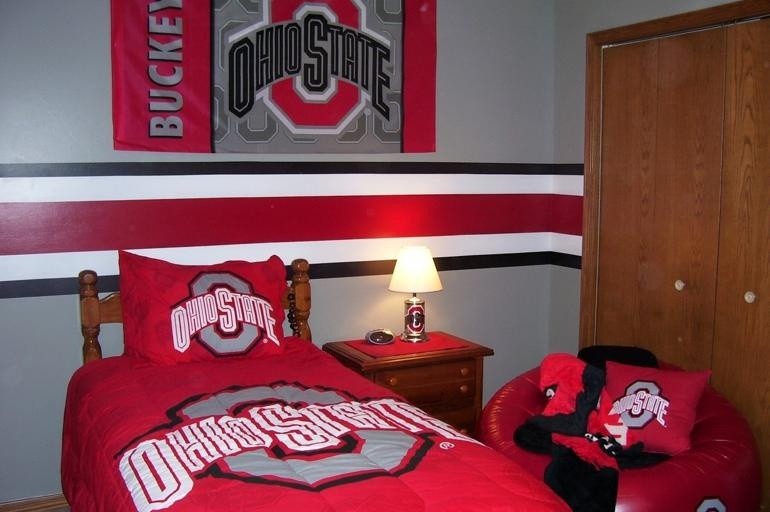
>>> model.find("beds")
[58,267,573,512]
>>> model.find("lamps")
[386,245,443,343]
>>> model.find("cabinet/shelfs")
[577,0,770,512]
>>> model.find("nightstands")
[322,330,495,442]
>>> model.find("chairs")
[476,363,764,512]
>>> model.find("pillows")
[115,246,290,370]
[603,361,714,457]
